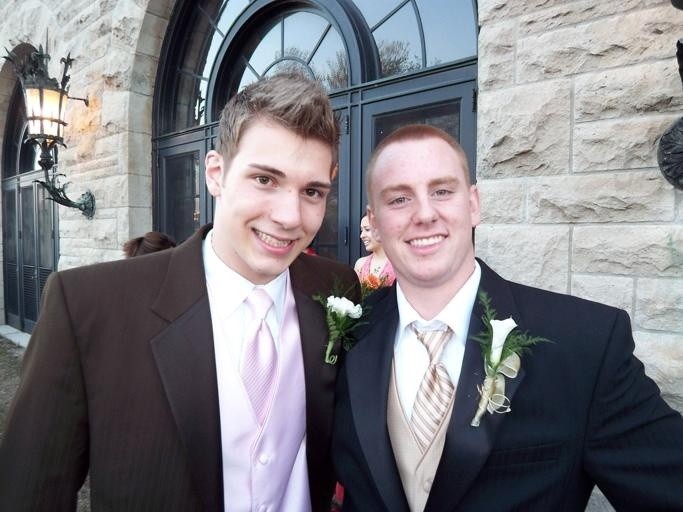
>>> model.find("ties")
[407,322,455,453]
[239,289,279,429]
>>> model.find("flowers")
[462,287,557,388]
[309,269,393,367]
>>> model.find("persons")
[352,212,397,300]
[122,230,179,258]
[0,70,359,511]
[325,121,682,511]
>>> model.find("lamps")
[0,41,96,219]
